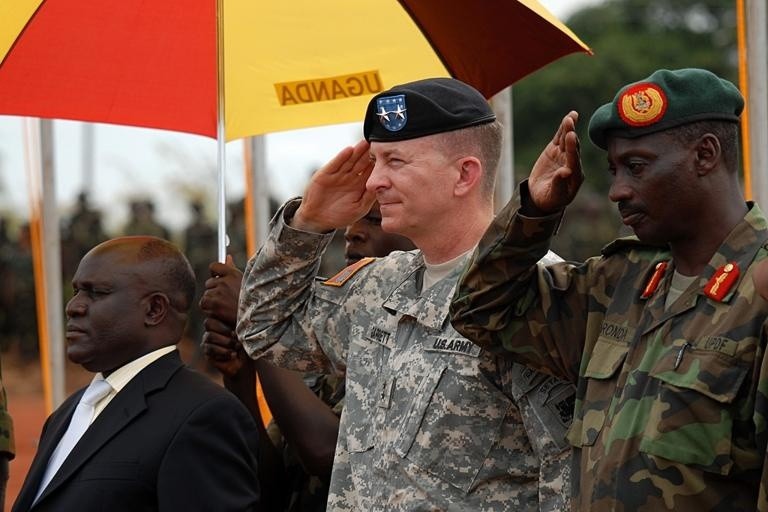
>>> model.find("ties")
[30,378,113,508]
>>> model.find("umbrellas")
[0,2,595,278]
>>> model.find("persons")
[197,199,417,511]
[10,235,262,511]
[233,76,573,511]
[448,68,768,511]
[0,186,285,361]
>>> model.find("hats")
[363,77,497,146]
[587,66,746,151]
